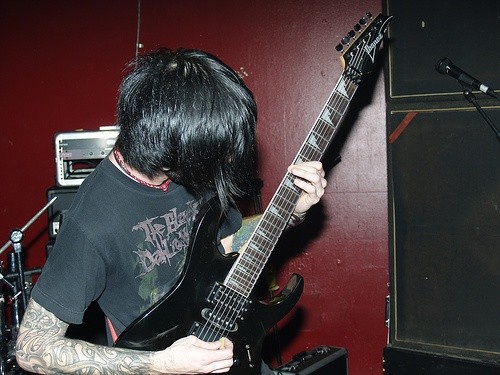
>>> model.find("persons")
[16,47,327,375]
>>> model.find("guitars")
[112,10,397,375]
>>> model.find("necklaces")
[113,148,171,192]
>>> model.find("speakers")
[382,0,500,375]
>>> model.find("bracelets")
[287,213,304,227]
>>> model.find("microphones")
[434,57,494,97]
